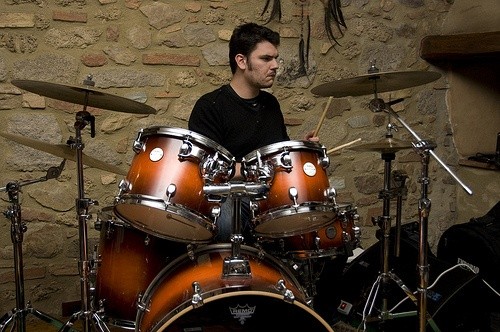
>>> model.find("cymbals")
[311,69,444,99]
[348,136,434,152]
[1,130,128,176]
[9,79,157,114]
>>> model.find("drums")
[250,201,363,261]
[241,138,334,217]
[112,125,237,246]
[89,205,204,332]
[135,233,334,332]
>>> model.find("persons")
[186,23,321,243]
